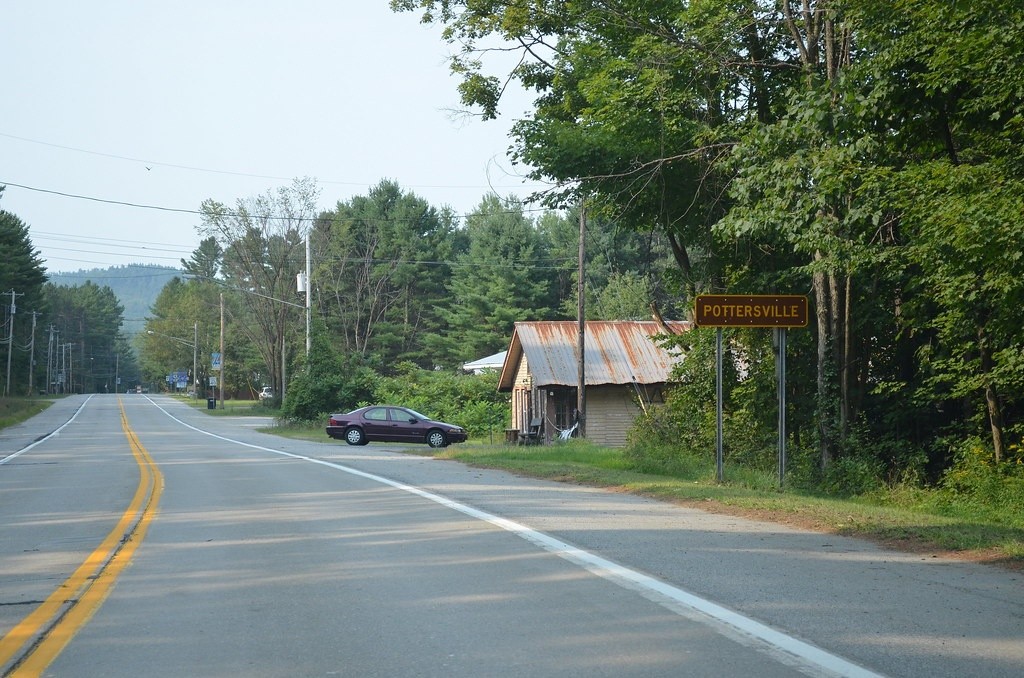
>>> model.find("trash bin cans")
[206,398,216,409]
[504,429,518,443]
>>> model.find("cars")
[326,405,472,448]
[128,386,137,394]
[258,386,273,402]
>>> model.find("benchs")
[517,417,544,446]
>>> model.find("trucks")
[137,385,142,394]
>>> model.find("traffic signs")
[172,372,187,382]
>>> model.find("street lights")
[70,358,94,393]
[147,330,199,401]
[182,273,313,379]
